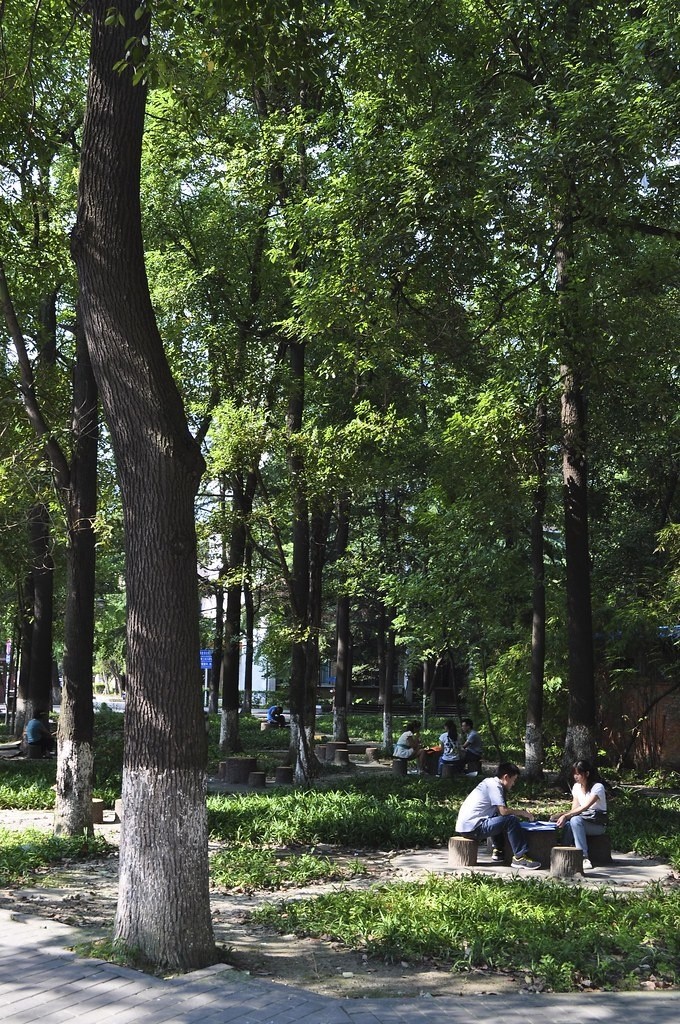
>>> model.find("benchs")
[350,704,470,718]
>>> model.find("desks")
[261,722,279,733]
[503,824,557,869]
[226,757,257,784]
[326,742,347,761]
[418,749,443,774]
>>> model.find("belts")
[396,744,411,749]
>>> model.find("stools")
[441,763,454,778]
[334,748,349,764]
[92,798,104,823]
[487,836,497,855]
[27,745,42,760]
[366,747,380,764]
[218,762,226,779]
[549,846,583,879]
[276,767,293,783]
[115,799,121,822]
[448,836,478,866]
[587,833,612,866]
[317,746,326,761]
[392,758,407,780]
[248,772,265,788]
[469,761,482,775]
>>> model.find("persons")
[27,710,55,759]
[267,706,287,727]
[436,719,461,777]
[392,720,427,774]
[549,760,609,869]
[455,762,541,870]
[460,717,483,774]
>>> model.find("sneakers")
[511,854,541,869]
[491,849,504,860]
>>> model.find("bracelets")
[564,815,567,820]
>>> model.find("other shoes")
[436,775,441,778]
[582,859,592,868]
[42,756,49,759]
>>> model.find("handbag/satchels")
[459,749,470,761]
[426,746,442,756]
